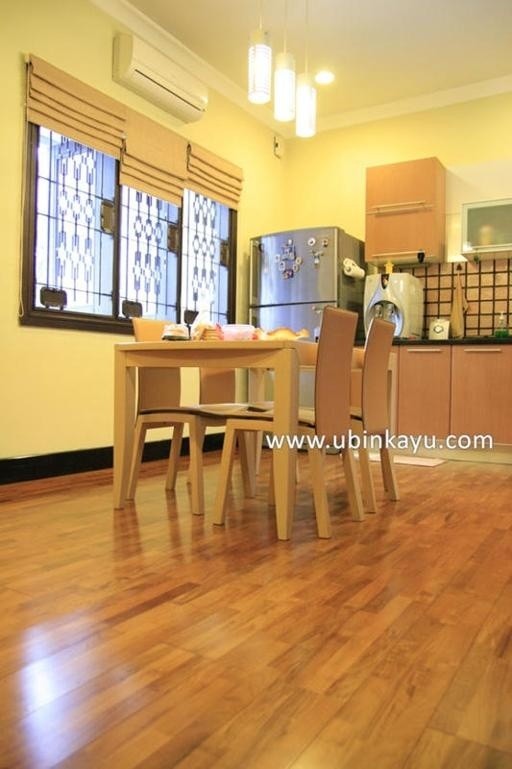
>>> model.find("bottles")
[478,223,492,246]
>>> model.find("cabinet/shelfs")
[397,340,451,448]
[450,342,509,451]
[364,152,447,264]
[459,197,512,257]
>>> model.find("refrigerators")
[246,224,365,458]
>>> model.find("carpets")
[344,447,449,468]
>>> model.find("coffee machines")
[360,272,425,341]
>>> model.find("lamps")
[274,0,298,125]
[241,0,273,105]
[295,0,319,143]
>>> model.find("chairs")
[289,315,399,516]
[123,312,259,520]
[213,307,362,543]
[190,320,303,501]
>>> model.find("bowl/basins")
[221,323,256,342]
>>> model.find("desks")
[109,337,397,514]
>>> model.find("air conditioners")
[110,29,212,130]
